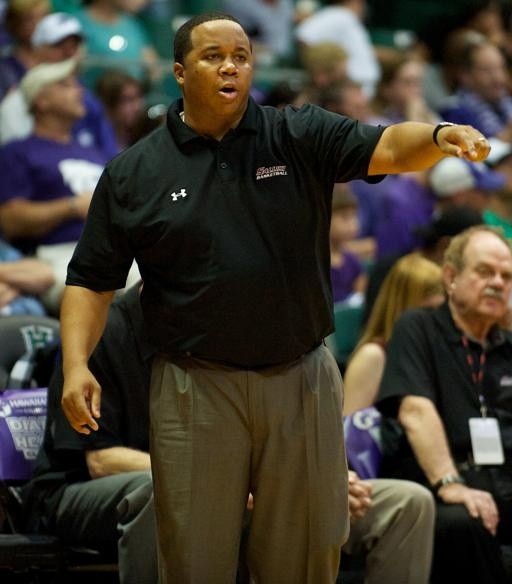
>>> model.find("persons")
[0,1,511,584]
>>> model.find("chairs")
[0,303,384,584]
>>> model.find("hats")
[483,135,511,164]
[429,153,476,197]
[33,11,85,45]
[20,57,79,112]
